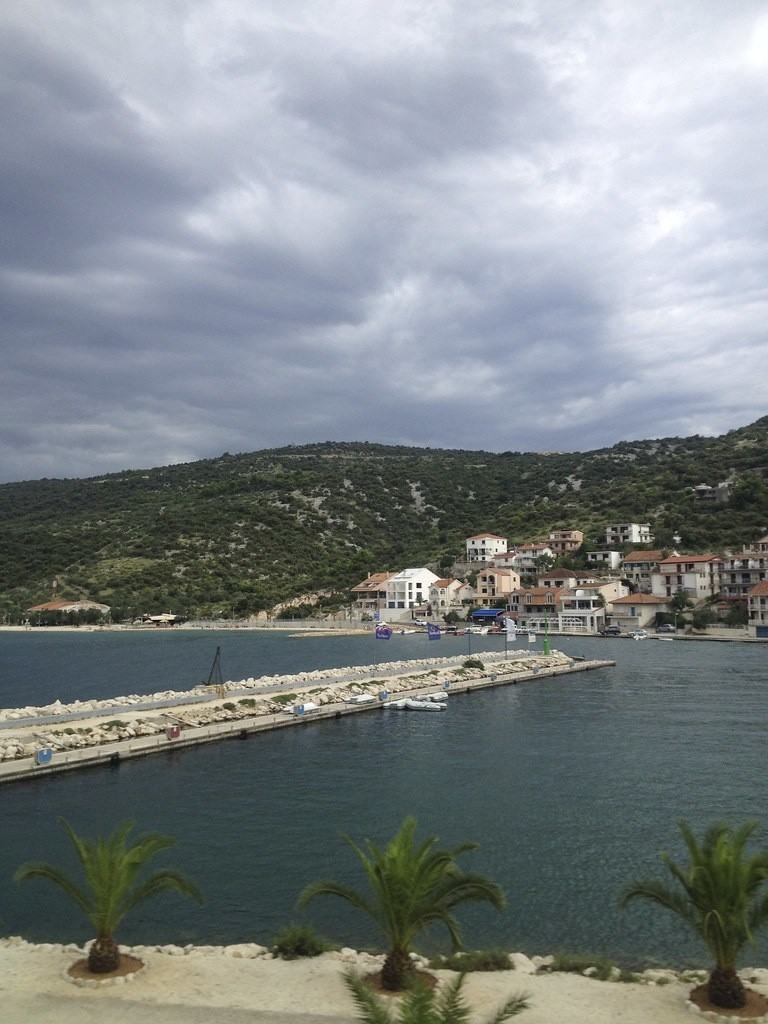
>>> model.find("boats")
[445,627,465,635]
[415,692,449,702]
[382,698,412,710]
[405,701,447,711]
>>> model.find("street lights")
[675,612,678,636]
[468,612,473,659]
[505,607,508,660]
[233,607,235,621]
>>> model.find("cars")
[361,616,372,621]
[656,624,675,632]
[627,629,647,636]
[600,626,621,635]
[416,620,427,625]
[466,625,482,632]
